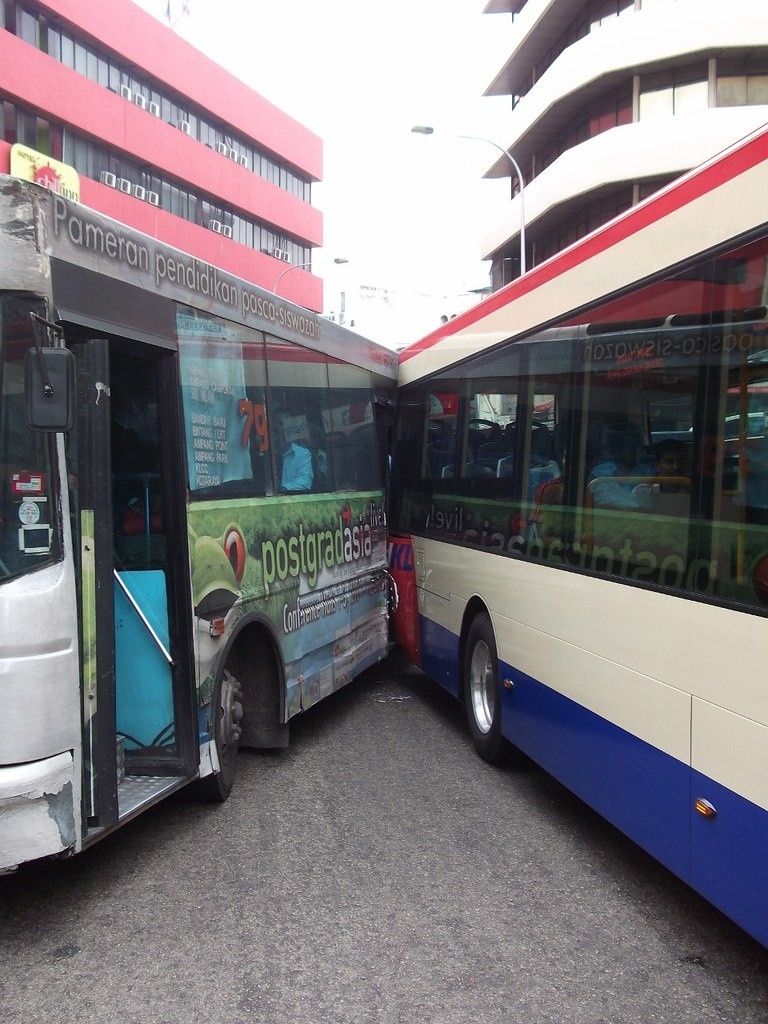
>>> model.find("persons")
[0,223,768,618]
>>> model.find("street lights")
[272,255,348,315]
[410,120,529,274]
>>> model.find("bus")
[0,171,391,885]
[393,129,767,950]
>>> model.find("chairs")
[406,412,729,557]
